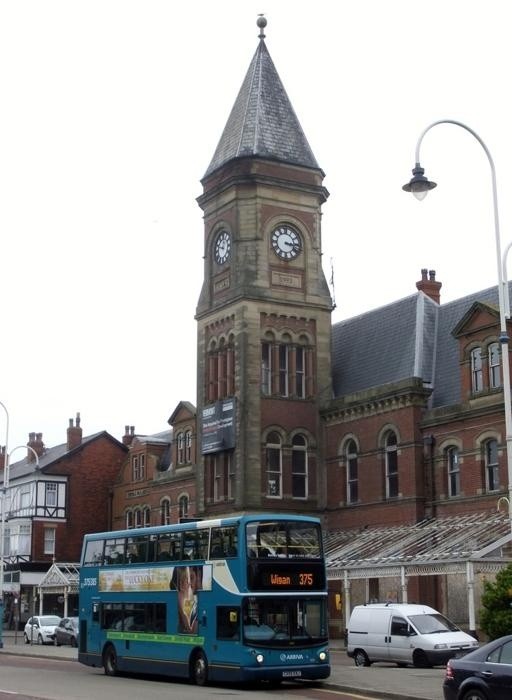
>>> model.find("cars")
[23,614,62,646]
[442,634,512,700]
[53,616,78,648]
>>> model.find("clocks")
[270,224,302,262]
[214,231,231,265]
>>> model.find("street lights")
[0,445,41,648]
[400,119,511,492]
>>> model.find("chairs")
[106,546,268,564]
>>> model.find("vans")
[347,601,480,667]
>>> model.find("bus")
[76,512,331,686]
